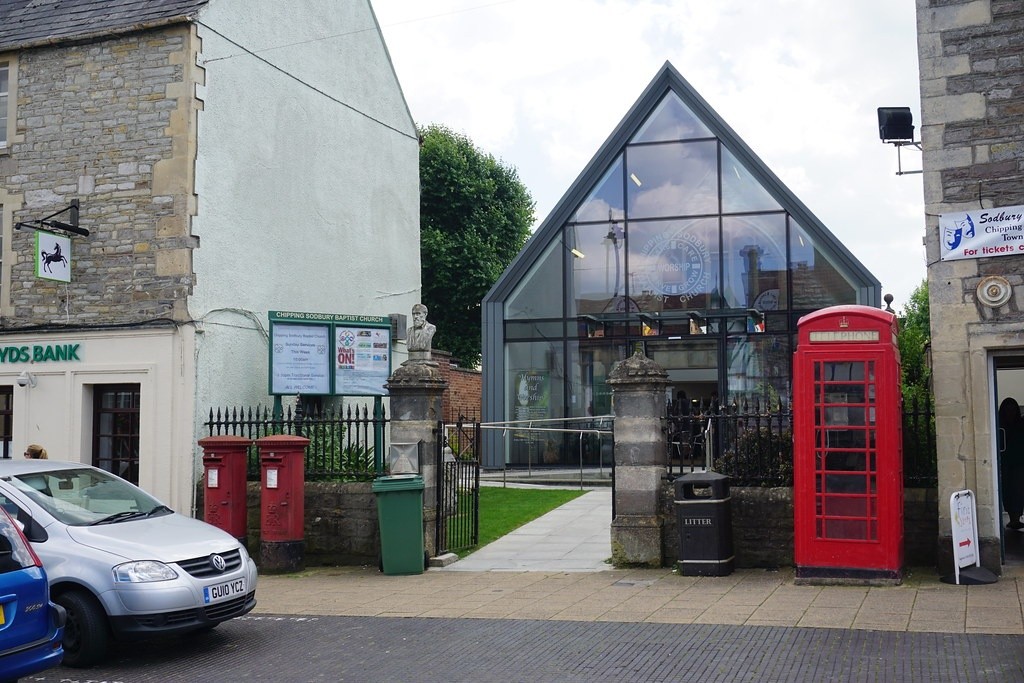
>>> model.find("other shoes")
[1006,521,1024,529]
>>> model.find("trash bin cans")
[674,471,735,578]
[372,474,425,575]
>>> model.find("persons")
[407,304,436,352]
[672,390,696,416]
[24,445,49,482]
[711,391,719,415]
[999,398,1024,528]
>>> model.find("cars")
[0,504,68,683]
[0,458,258,669]
